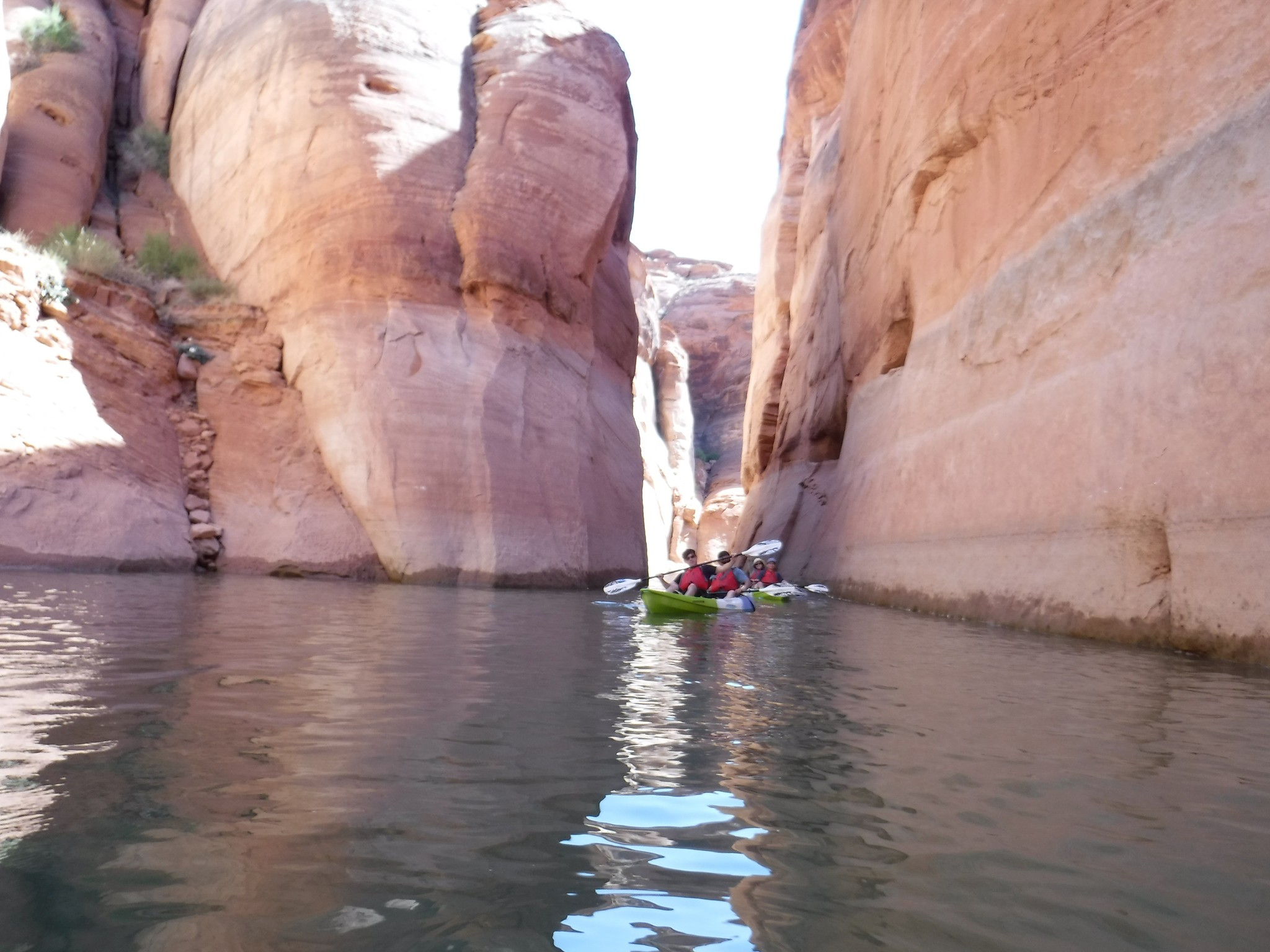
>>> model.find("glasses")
[685,555,695,561]
[718,560,730,564]
[755,563,761,565]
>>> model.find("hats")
[752,558,766,566]
[767,558,777,563]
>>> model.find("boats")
[640,589,790,616]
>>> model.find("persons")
[700,551,751,599]
[749,558,797,590]
[655,551,738,596]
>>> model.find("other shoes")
[673,589,683,596]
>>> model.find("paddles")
[701,582,795,597]
[603,538,784,597]
[797,582,829,595]
